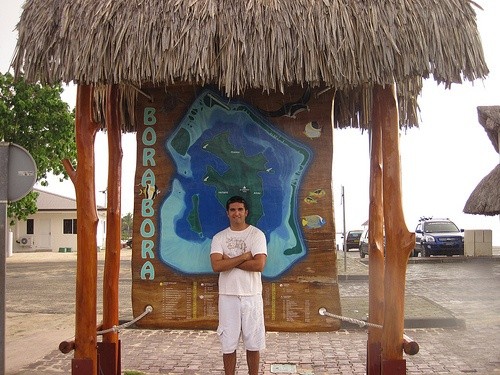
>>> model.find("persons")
[209,197,268,375]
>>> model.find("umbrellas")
[463,161,500,216]
[477,104,500,155]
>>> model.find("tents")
[8,0,489,375]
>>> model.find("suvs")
[359,225,386,258]
[411,216,465,257]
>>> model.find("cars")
[341,230,364,252]
